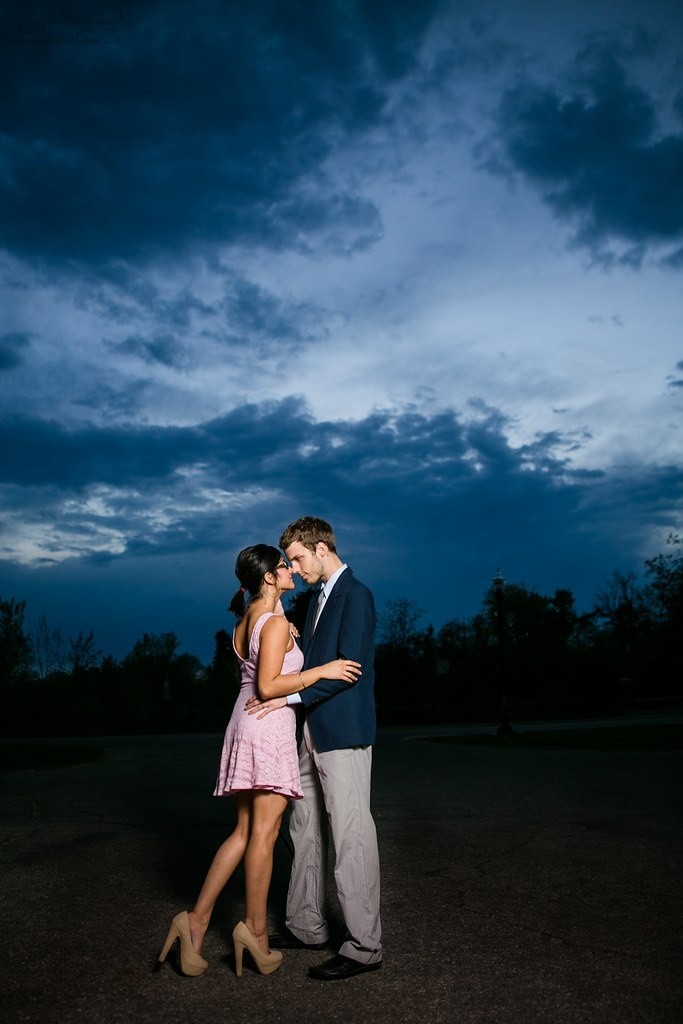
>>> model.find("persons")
[157,544,365,977]
[245,514,386,981]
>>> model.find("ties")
[310,589,324,640]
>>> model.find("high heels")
[233,921,283,977]
[158,911,208,976]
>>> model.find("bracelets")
[298,672,308,690]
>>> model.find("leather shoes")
[269,927,328,950]
[309,954,382,981]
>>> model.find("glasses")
[275,561,288,569]
